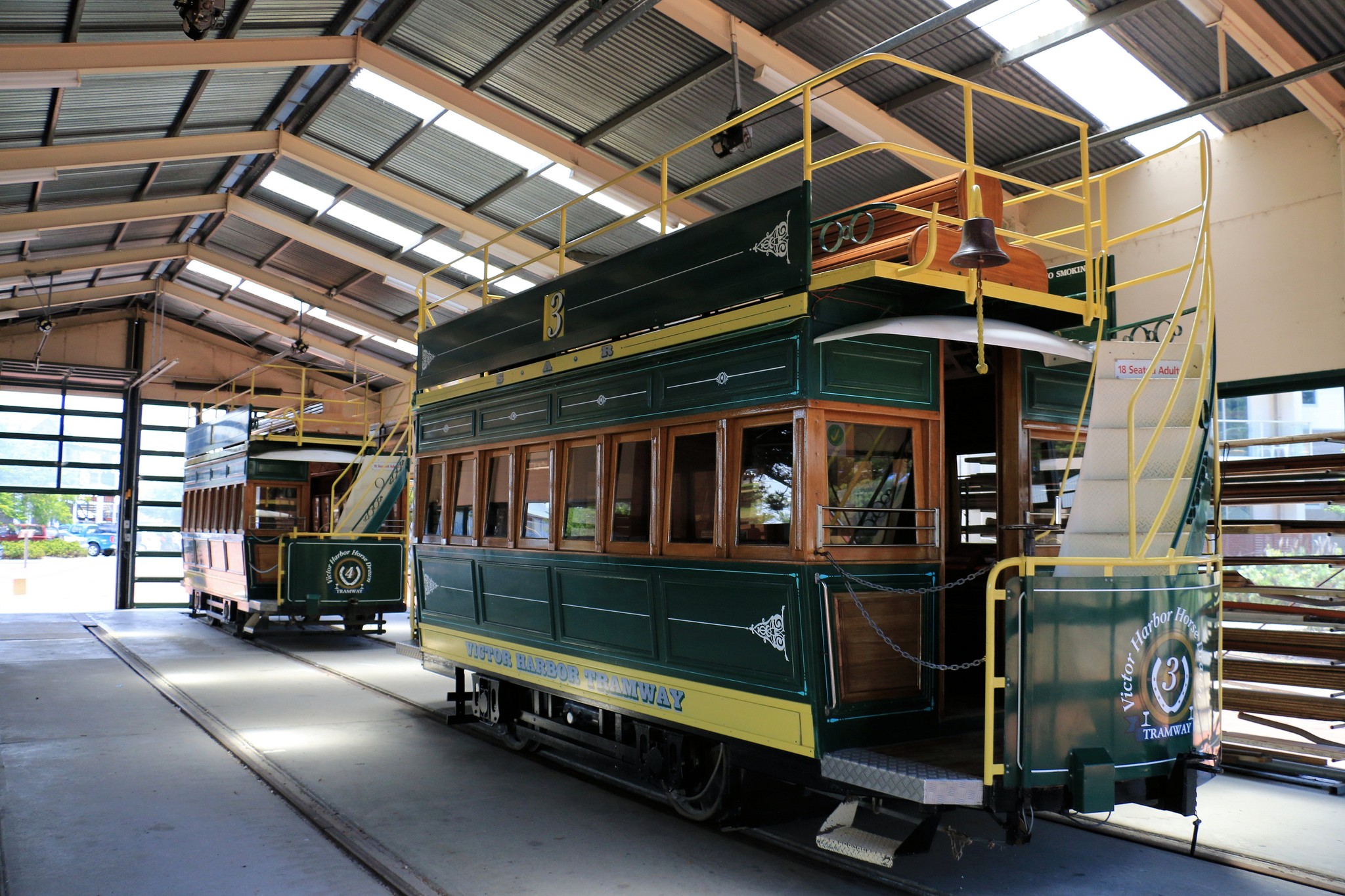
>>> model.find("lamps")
[711,16,753,158]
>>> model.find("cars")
[0,521,117,557]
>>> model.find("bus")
[401,53,1223,869]
[180,363,416,637]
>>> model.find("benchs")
[249,388,370,436]
[812,168,1050,295]
